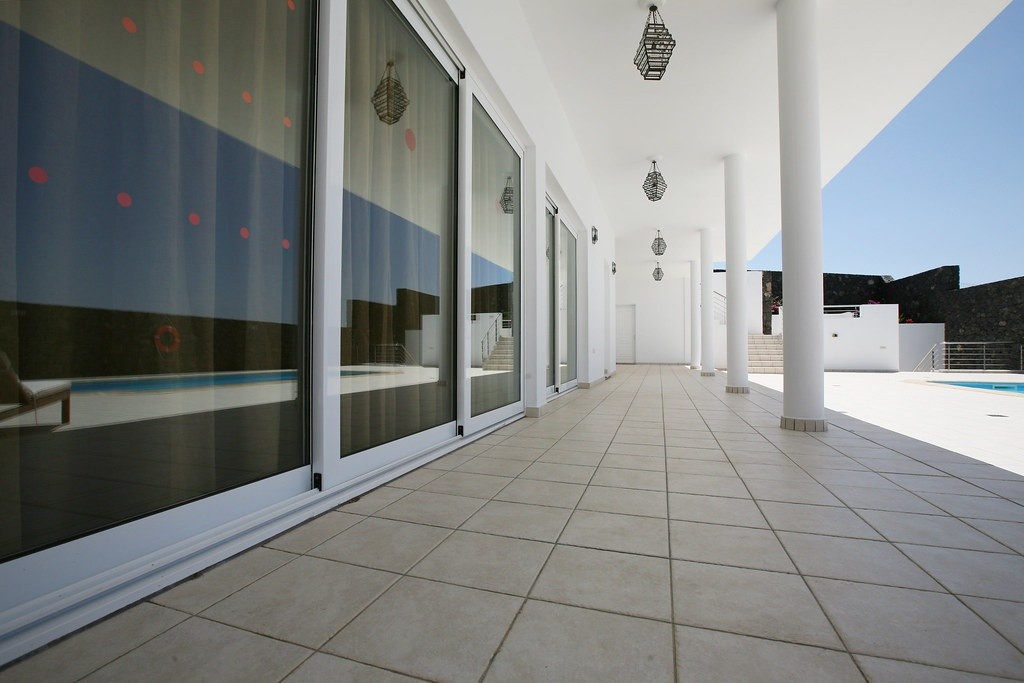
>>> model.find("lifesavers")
[155,325,180,354]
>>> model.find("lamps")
[372,58,413,127]
[634,4,676,83]
[652,262,664,282]
[501,175,517,214]
[642,161,668,203]
[651,230,667,256]
